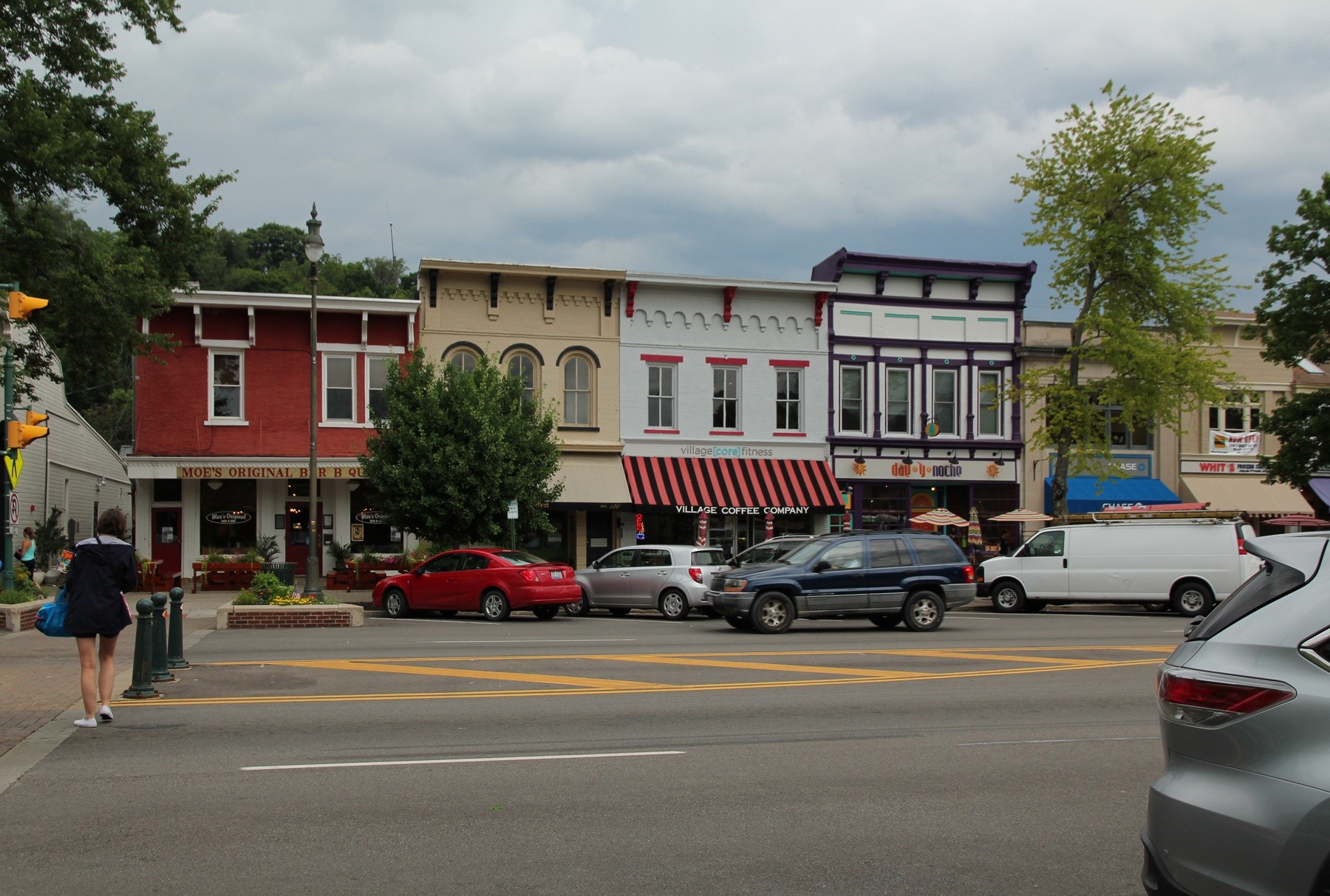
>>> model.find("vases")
[344,559,422,593]
[192,560,261,594]
[136,559,164,594]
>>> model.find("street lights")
[301,201,326,602]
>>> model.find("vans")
[974,513,1266,617]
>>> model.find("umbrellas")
[906,507,969,527]
[1262,513,1330,533]
[967,505,982,545]
[986,508,1053,546]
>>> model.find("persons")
[65,508,138,727]
[16,527,41,590]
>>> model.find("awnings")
[1178,478,1315,517]
[623,455,845,506]
[1044,477,1182,515]
[1307,478,1330,508]
[538,453,632,503]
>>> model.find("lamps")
[853,446,866,464]
[947,447,959,465]
[993,448,1005,466]
[96,476,107,492]
[900,447,914,465]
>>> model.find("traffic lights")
[8,291,49,320]
[7,421,50,448]
[26,410,49,426]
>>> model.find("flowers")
[134,548,151,589]
[351,545,430,583]
[196,543,264,588]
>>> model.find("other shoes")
[100,706,113,720]
[73,715,97,727]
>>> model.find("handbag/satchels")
[120,591,132,619]
[34,587,68,638]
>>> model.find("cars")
[1140,530,1330,896]
[562,544,733,621]
[372,547,582,622]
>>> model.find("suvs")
[724,532,819,568]
[704,528,977,634]
